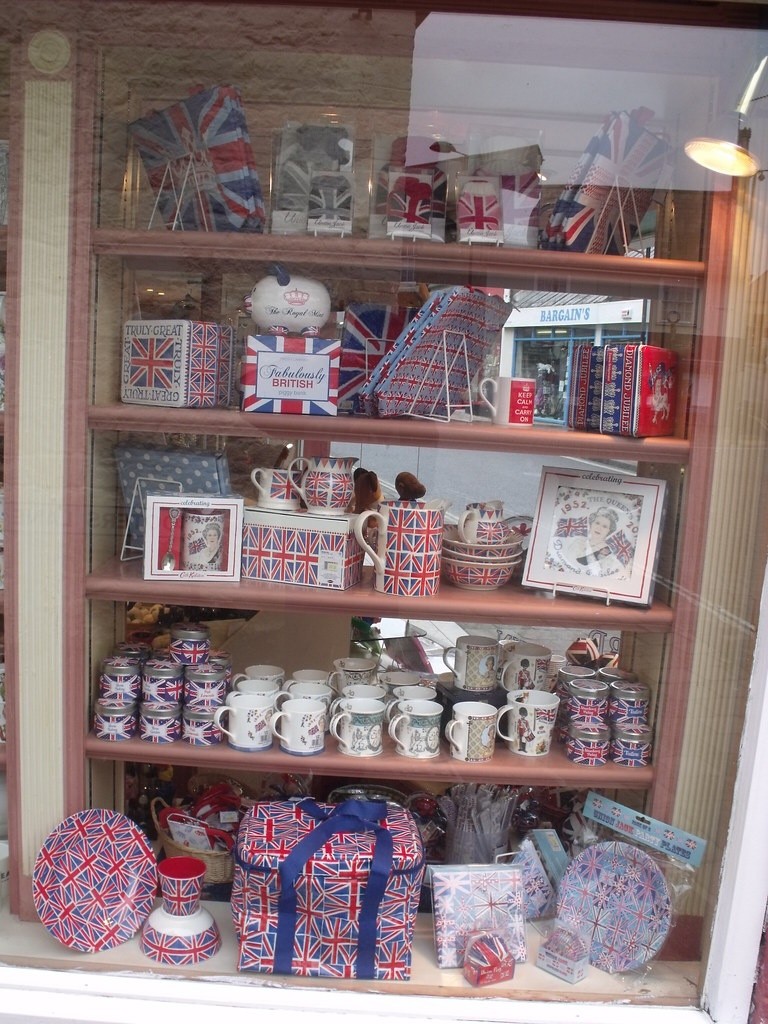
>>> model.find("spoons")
[161,507,181,570]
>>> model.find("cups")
[251,467,302,510]
[444,822,508,864]
[498,639,520,673]
[497,641,551,691]
[443,634,498,691]
[181,513,223,571]
[479,376,536,427]
[157,856,207,915]
[213,657,443,760]
[354,500,445,597]
[496,689,561,756]
[445,701,498,762]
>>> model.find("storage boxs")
[241,505,367,590]
[120,319,237,406]
[114,440,231,552]
[143,490,246,582]
[522,465,671,609]
[568,344,680,440]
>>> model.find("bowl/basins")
[441,524,523,591]
[138,902,222,966]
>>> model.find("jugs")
[287,456,359,516]
[457,499,512,544]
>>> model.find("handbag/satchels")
[231,800,426,980]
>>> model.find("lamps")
[683,52,768,179]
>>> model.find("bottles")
[91,621,232,745]
[545,655,653,768]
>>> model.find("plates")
[32,808,157,953]
[556,841,673,973]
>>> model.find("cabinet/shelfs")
[89,45,734,916]
